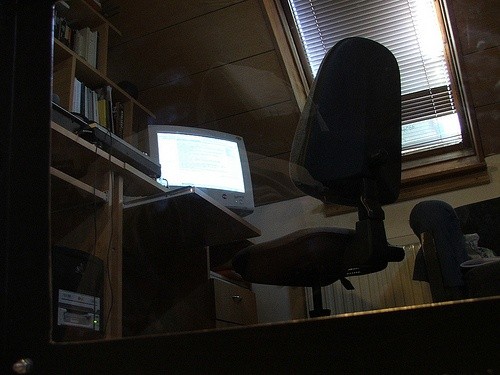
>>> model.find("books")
[53,12,127,144]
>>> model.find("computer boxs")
[53,246,107,341]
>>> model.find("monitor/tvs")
[140,125,255,218]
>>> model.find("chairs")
[230,36,405,315]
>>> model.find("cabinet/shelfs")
[0,0,262,342]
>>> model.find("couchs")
[409,196,499,303]
